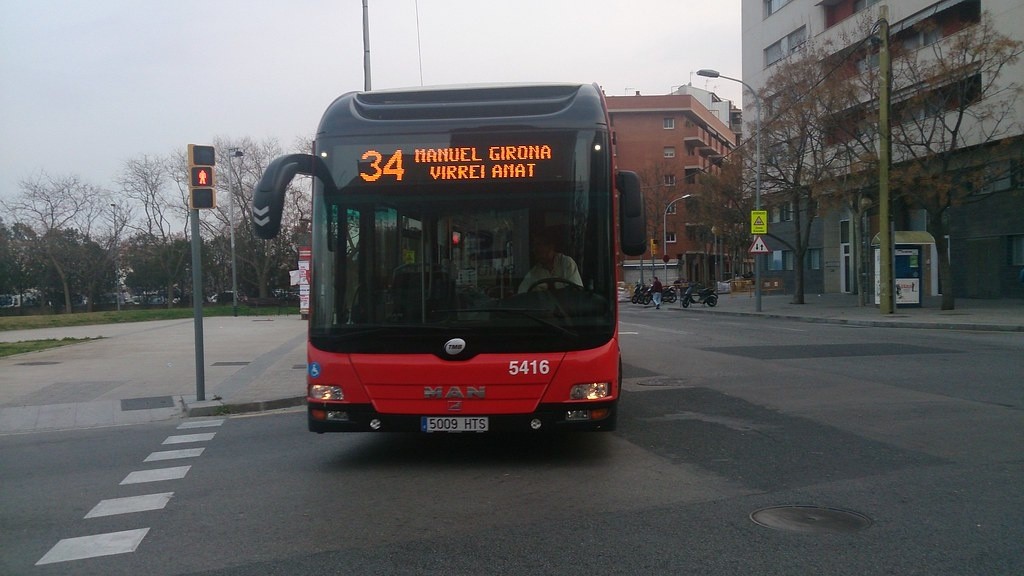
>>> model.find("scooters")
[682,279,718,308]
[631,277,678,305]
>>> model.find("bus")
[250,84,649,434]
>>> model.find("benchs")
[749,278,786,297]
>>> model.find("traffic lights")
[187,144,216,209]
[651,239,658,258]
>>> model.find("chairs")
[393,252,514,311]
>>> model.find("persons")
[517,230,584,295]
[651,277,662,309]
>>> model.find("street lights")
[111,203,121,312]
[663,193,693,288]
[696,69,764,313]
[711,225,718,283]
[227,146,244,314]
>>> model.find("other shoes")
[656,308,659,309]
[657,304,659,307]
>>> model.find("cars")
[0,284,301,309]
